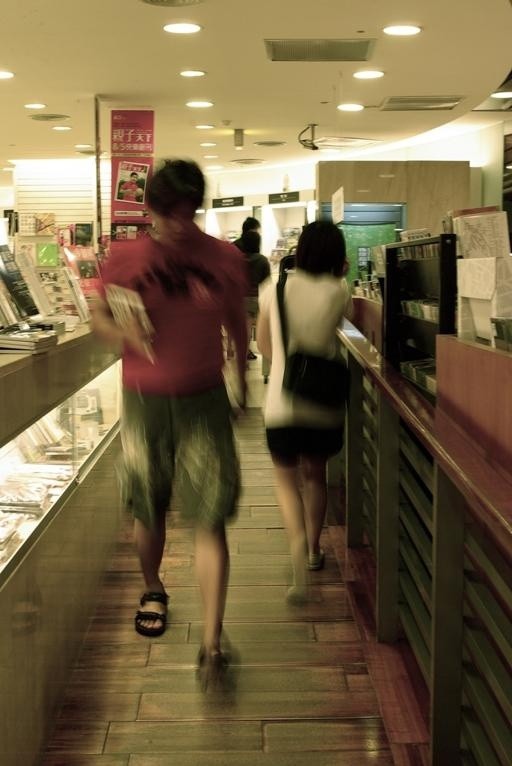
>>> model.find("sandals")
[134,582,167,637]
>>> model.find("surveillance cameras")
[304,144,319,150]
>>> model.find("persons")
[256,221,355,604]
[227,217,272,360]
[92,160,248,666]
[119,172,138,201]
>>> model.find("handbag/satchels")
[281,352,351,409]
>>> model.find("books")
[103,281,159,364]
[0,319,57,354]
[400,358,435,395]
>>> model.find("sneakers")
[305,548,325,570]
